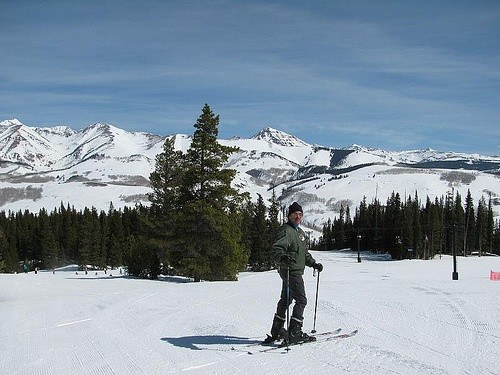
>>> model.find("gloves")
[282,255,293,264]
[313,263,322,271]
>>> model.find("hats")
[288,201,303,213]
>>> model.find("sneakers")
[270,327,292,340]
[289,329,312,340]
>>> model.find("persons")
[270,202,322,341]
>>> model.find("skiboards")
[232,328,358,355]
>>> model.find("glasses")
[290,211,303,216]
